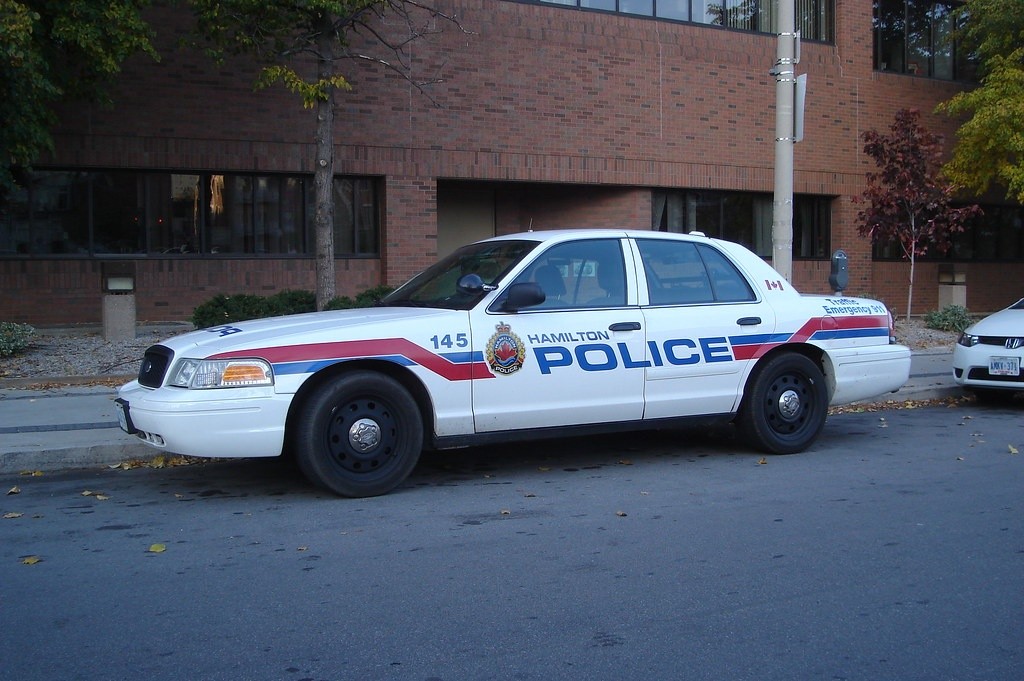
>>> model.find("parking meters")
[829,249,848,296]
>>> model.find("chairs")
[533,265,567,309]
[582,258,626,307]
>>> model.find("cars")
[953,296,1024,402]
[115,228,913,498]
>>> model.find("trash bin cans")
[102,260,137,340]
[937,262,967,312]
